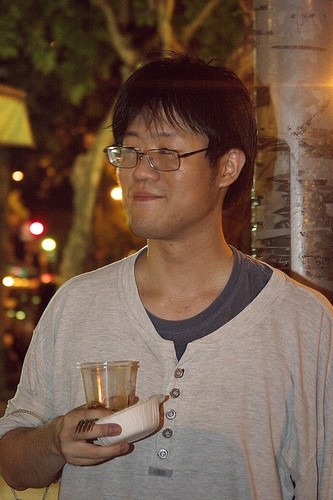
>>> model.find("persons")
[1,51,332,500]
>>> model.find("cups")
[75,360,140,412]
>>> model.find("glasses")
[104,141,211,172]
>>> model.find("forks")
[74,417,99,434]
